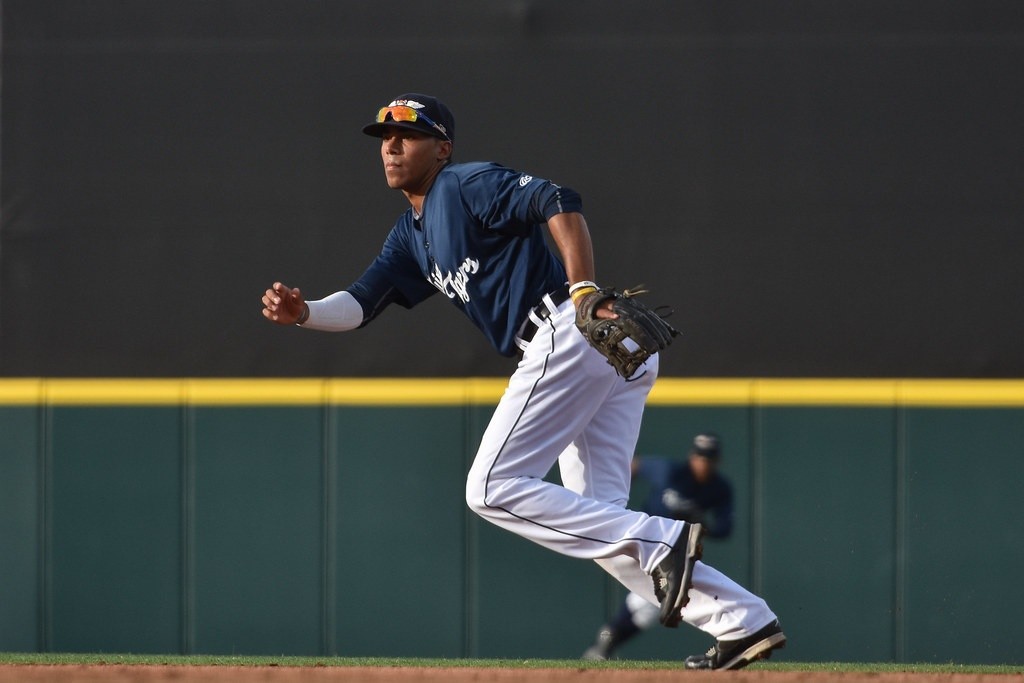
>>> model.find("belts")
[521,284,572,342]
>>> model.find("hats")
[361,94,455,144]
[693,432,723,458]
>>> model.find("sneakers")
[648,519,703,626]
[686,618,786,673]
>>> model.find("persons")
[580,430,737,660]
[263,94,787,671]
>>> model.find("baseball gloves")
[572,285,685,378]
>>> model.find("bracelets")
[296,306,305,323]
[569,281,600,303]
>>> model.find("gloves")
[663,488,684,510]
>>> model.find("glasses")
[376,105,454,143]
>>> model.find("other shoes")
[582,645,606,660]
